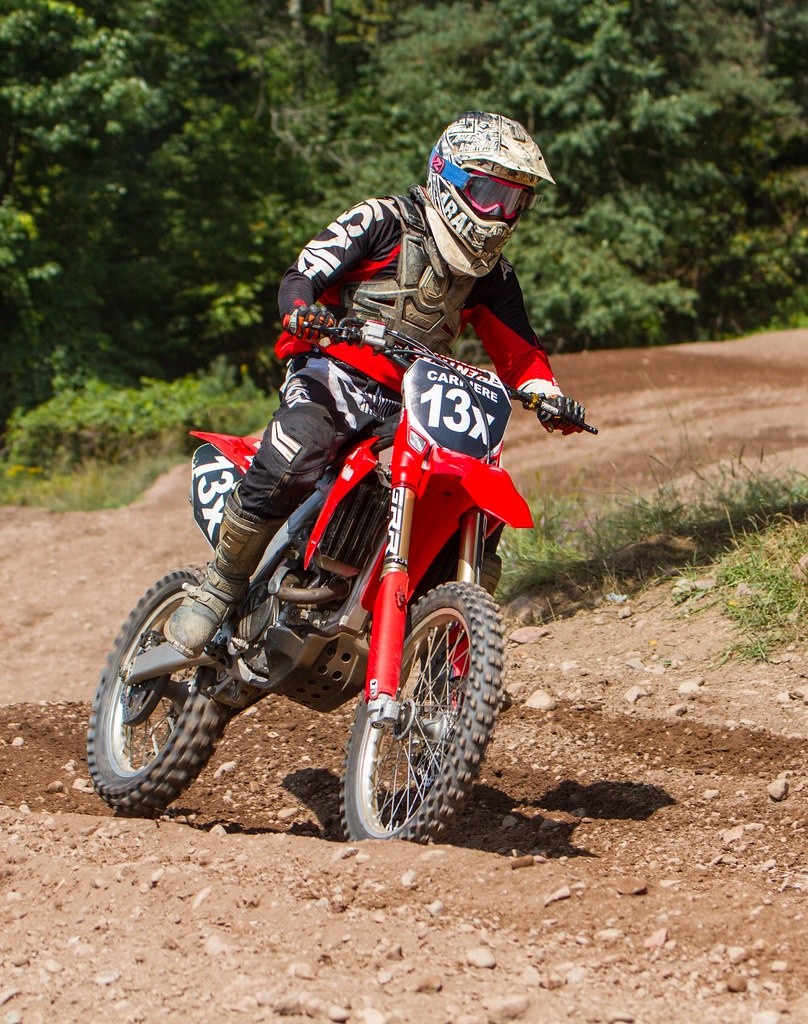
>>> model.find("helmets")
[423,109,559,259]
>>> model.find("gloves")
[288,302,336,344]
[539,396,585,437]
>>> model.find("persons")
[163,112,586,713]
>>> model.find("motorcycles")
[86,315,598,843]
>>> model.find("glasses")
[427,148,529,221]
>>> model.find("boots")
[414,551,514,716]
[162,478,295,660]
